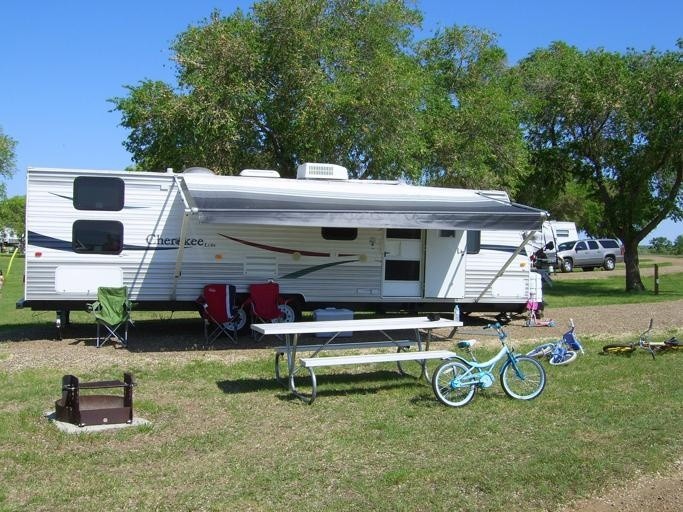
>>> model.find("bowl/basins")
[428,313,441,321]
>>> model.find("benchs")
[297,348,456,403]
[274,340,423,392]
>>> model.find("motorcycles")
[530,240,553,281]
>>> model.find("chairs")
[195,283,245,346]
[91,285,133,348]
[246,283,293,343]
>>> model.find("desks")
[249,316,463,391]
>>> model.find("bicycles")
[432,319,546,408]
[525,318,584,365]
[603,319,683,360]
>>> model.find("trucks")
[532,220,578,251]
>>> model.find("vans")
[554,239,623,272]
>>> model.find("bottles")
[454,305,459,322]
